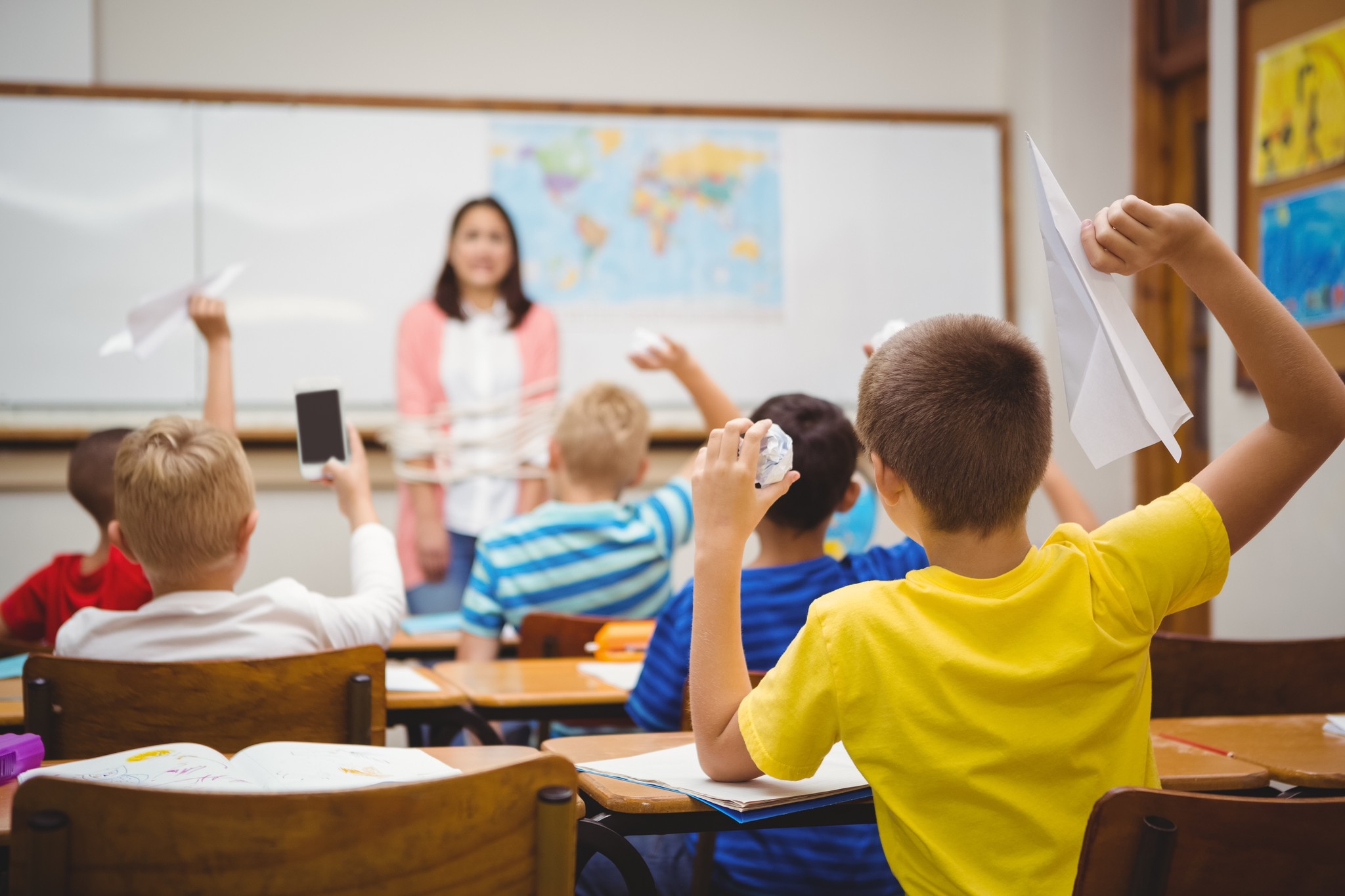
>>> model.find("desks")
[1152,713,1345,788]
[0,673,466,745]
[542,732,1271,895]
[437,656,630,723]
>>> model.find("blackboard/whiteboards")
[1,83,1019,444]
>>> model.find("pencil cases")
[594,614,657,661]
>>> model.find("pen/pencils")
[582,641,653,652]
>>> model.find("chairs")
[0,614,1345,896]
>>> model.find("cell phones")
[295,389,350,483]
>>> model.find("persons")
[397,198,559,747]
[0,292,1106,896]
[689,195,1345,896]
